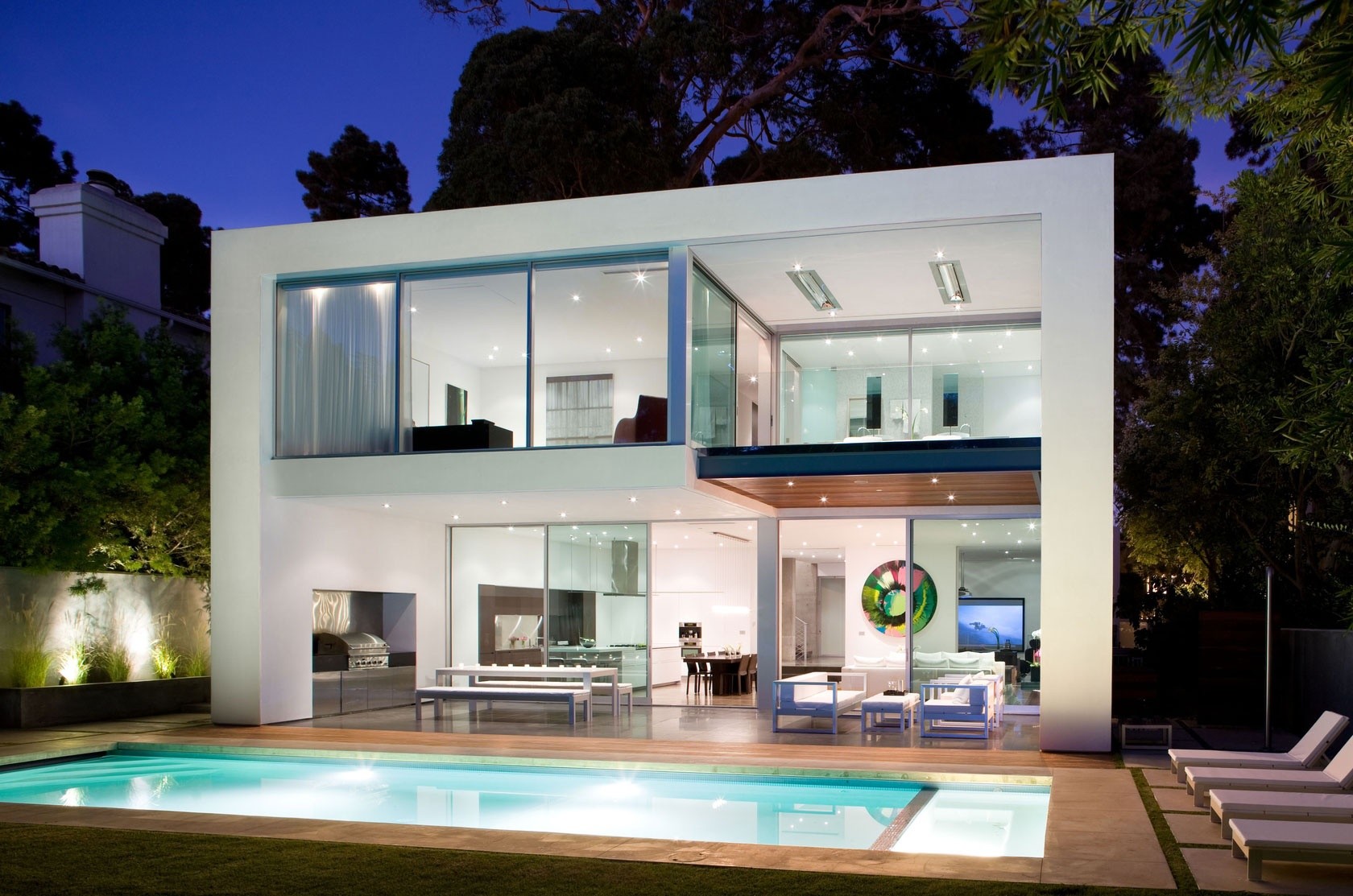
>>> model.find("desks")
[368,422,514,452]
[431,664,619,723]
[683,652,752,694]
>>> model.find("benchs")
[769,669,869,738]
[413,684,593,727]
[1117,717,1174,752]
[477,679,635,718]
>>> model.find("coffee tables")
[858,687,920,737]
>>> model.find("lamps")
[931,258,974,309]
[782,265,843,314]
[712,531,751,546]
[958,551,973,599]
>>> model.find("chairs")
[1184,734,1353,807]
[611,394,668,443]
[1226,816,1353,883]
[682,642,758,697]
[1166,709,1350,784]
[918,670,1006,740]
[1208,788,1353,839]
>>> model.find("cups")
[682,634,685,637]
[694,633,697,638]
[725,649,741,657]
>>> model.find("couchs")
[840,649,1007,698]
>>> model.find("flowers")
[894,405,930,438]
[519,636,529,641]
[507,635,518,642]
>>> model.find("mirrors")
[941,372,961,428]
[862,374,885,432]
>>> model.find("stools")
[548,654,622,682]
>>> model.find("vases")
[510,643,515,648]
[521,643,525,648]
